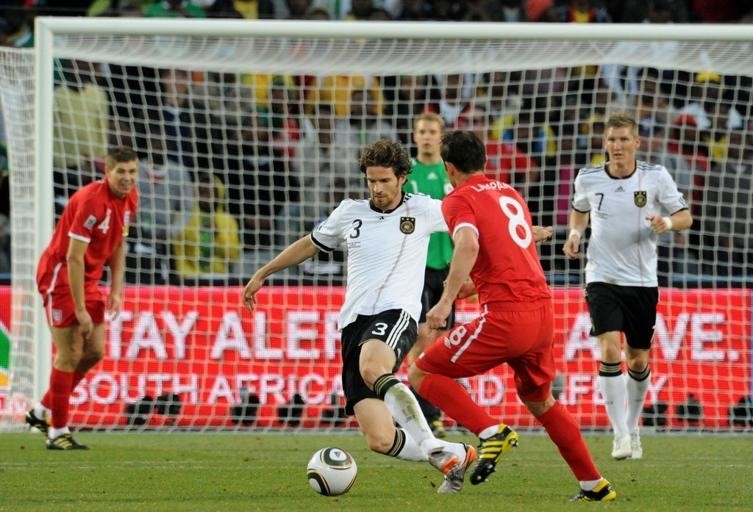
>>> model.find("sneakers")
[426,423,518,493]
[570,479,616,502]
[26,408,87,450]
[612,433,642,459]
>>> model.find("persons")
[239,135,553,494]
[561,114,695,460]
[2,1,753,288]
[405,127,618,501]
[401,109,458,438]
[21,142,138,449]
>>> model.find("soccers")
[307,447,357,496]
